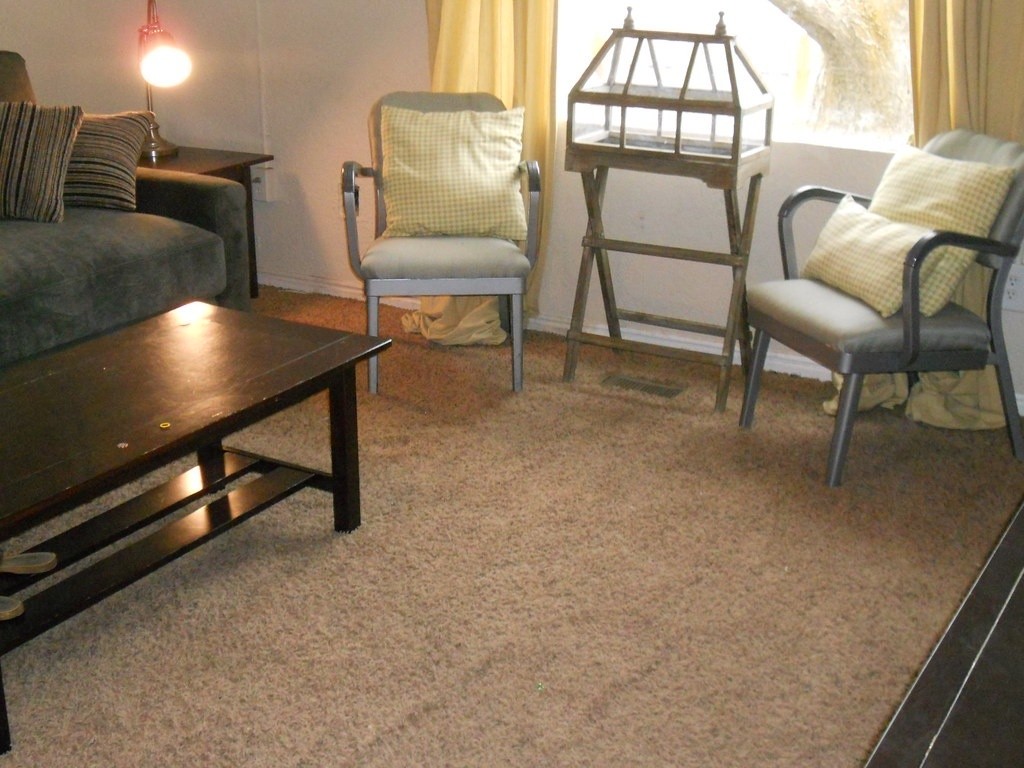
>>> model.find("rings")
[160,423,171,429]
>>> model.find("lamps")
[136,0,191,161]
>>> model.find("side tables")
[136,146,275,299]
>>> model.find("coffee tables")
[0,298,392,756]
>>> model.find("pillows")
[377,105,529,243]
[0,101,84,224]
[800,193,938,321]
[867,141,1015,319]
[63,111,155,211]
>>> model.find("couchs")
[0,48,250,363]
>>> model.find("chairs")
[739,127,1024,490]
[341,90,541,396]
